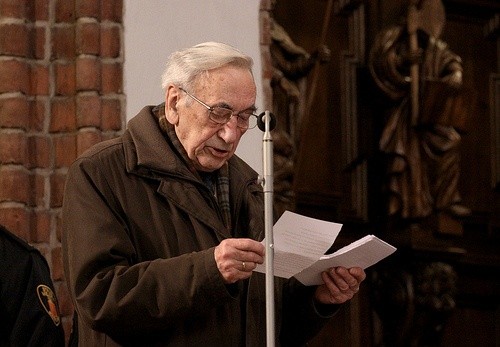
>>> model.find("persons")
[385,25,472,227]
[0,225,65,347]
[270,36,332,162]
[62,41,366,347]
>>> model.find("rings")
[242,261,246,271]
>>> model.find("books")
[293,234,396,286]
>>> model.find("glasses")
[178,87,258,129]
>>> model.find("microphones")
[257,110,277,141]
